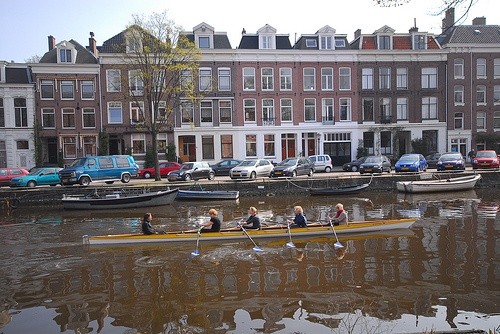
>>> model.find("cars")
[270,157,316,178]
[394,153,429,173]
[343,156,368,172]
[436,153,466,171]
[209,159,240,176]
[167,161,216,183]
[229,159,274,179]
[359,156,392,174]
[472,150,500,170]
[308,155,332,173]
[10,168,64,188]
[139,161,189,179]
[0,168,30,186]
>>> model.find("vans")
[58,155,139,187]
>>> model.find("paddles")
[190,228,200,257]
[237,223,265,252]
[286,219,296,248]
[329,216,344,249]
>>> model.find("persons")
[468,148,476,164]
[239,207,260,231]
[327,203,348,226]
[200,209,221,233]
[142,213,166,234]
[286,206,307,229]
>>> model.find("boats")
[82,218,417,245]
[397,173,481,193]
[176,190,240,200]
[285,173,374,195]
[60,187,180,210]
[81,227,420,251]
